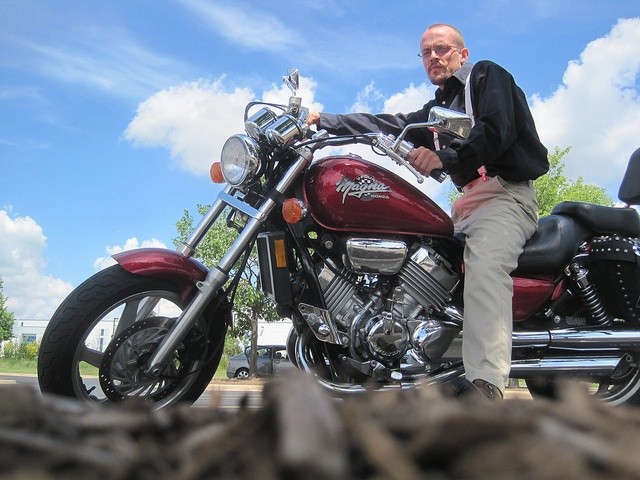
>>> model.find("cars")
[226,344,302,379]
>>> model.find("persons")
[295,22,552,401]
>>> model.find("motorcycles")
[37,67,640,413]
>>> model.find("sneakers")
[448,378,504,406]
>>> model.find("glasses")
[418,42,462,57]
